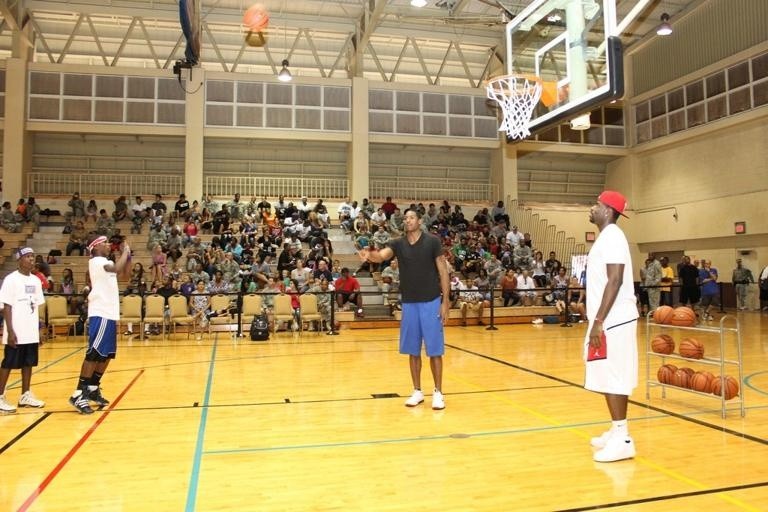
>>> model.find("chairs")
[35,292,322,341]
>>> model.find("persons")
[758,266,768,311]
[1,191,41,232]
[581,189,639,463]
[69,233,132,414]
[639,251,719,322]
[1,247,45,414]
[359,207,450,411]
[353,196,587,323]
[32,191,364,339]
[733,258,755,310]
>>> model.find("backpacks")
[251,314,269,340]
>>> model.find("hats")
[597,191,630,219]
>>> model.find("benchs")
[0,213,354,320]
[350,210,572,321]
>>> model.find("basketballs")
[243,6,268,31]
[648,304,740,401]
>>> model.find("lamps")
[655,12,672,37]
[277,58,292,82]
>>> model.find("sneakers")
[406,388,424,406]
[591,428,635,461]
[17,391,45,408]
[87,385,109,405]
[69,390,94,414]
[432,388,445,408]
[0,394,16,413]
